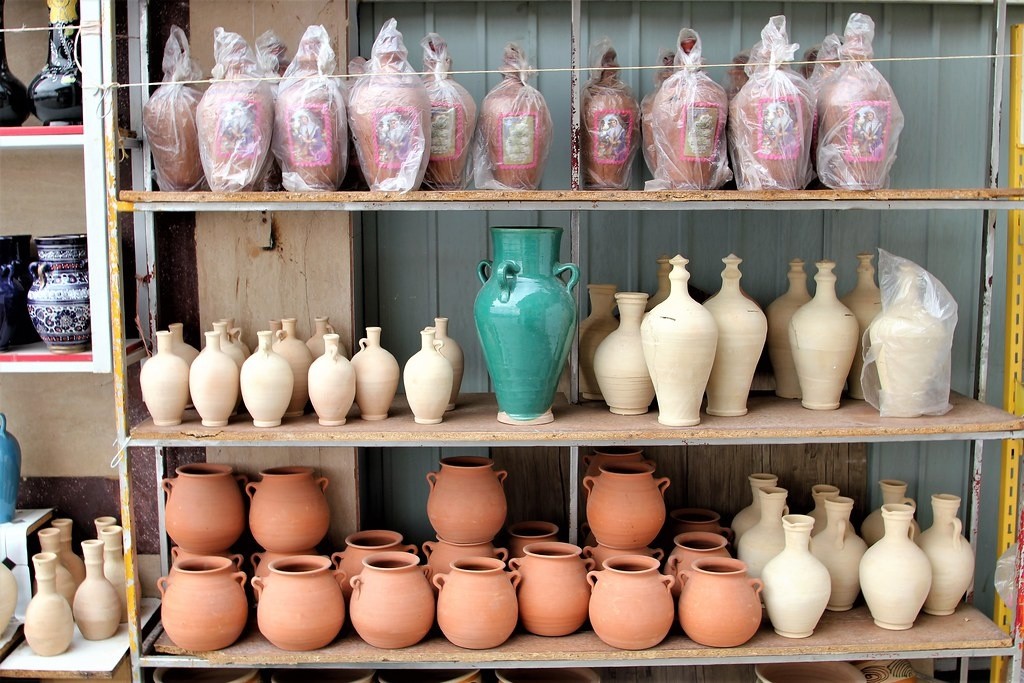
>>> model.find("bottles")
[140,34,554,191]
[0,413,21,524]
[1,515,141,656]
[1,0,83,128]
[474,225,945,427]
[138,316,465,428]
[0,233,91,354]
[578,12,898,191]
[733,474,976,640]
[156,446,765,653]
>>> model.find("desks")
[0,587,161,683]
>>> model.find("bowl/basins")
[153,666,261,683]
[755,660,867,683]
[495,666,601,683]
[378,668,483,683]
[271,669,375,683]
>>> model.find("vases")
[0,0,977,655]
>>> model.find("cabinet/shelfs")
[0,0,154,378]
[102,0,1024,683]
